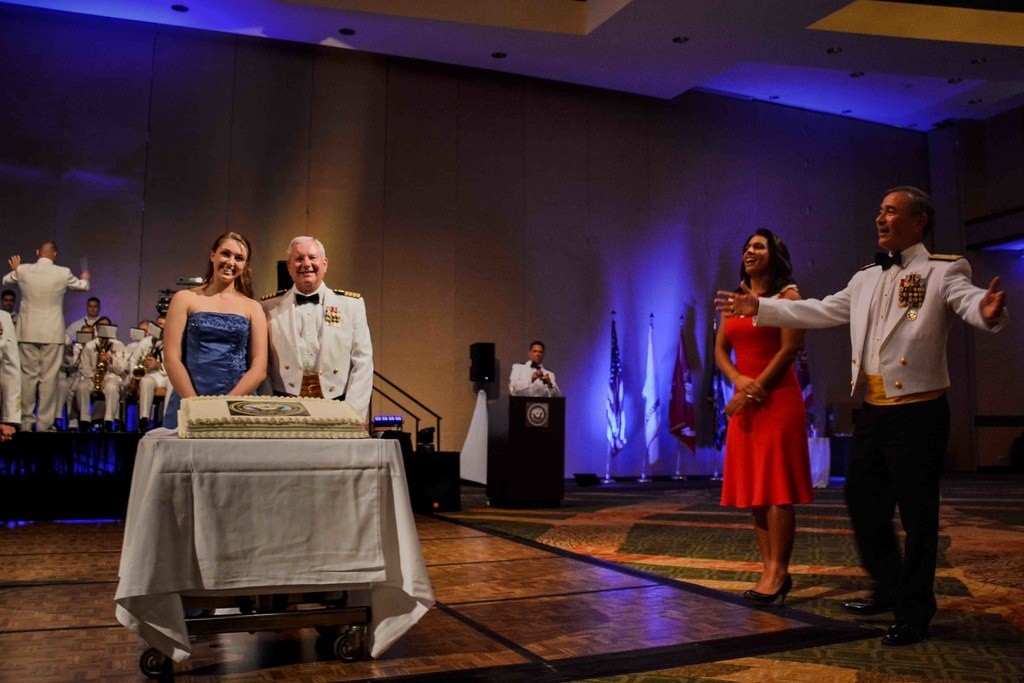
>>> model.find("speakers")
[277,260,293,294]
[469,342,496,384]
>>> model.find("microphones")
[537,366,544,377]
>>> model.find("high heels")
[745,573,792,603]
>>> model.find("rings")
[8,437,12,440]
[730,305,734,310]
[728,297,734,303]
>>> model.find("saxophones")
[93,338,106,392]
[125,338,158,401]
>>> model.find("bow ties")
[296,293,319,305]
[531,362,541,370]
[874,250,901,271]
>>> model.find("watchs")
[549,383,555,388]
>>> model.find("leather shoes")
[841,596,893,614]
[881,619,929,646]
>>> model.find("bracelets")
[746,395,752,398]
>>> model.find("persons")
[508,342,563,399]
[3,240,91,432]
[0,288,172,443]
[261,235,373,633]
[715,184,1010,645]
[714,228,816,606]
[164,232,267,634]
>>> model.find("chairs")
[71,386,165,431]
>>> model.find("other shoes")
[54,420,162,434]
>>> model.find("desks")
[806,434,852,489]
[114,429,436,677]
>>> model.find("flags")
[700,319,728,451]
[606,319,626,456]
[670,328,696,456]
[798,337,816,437]
[642,322,661,468]
